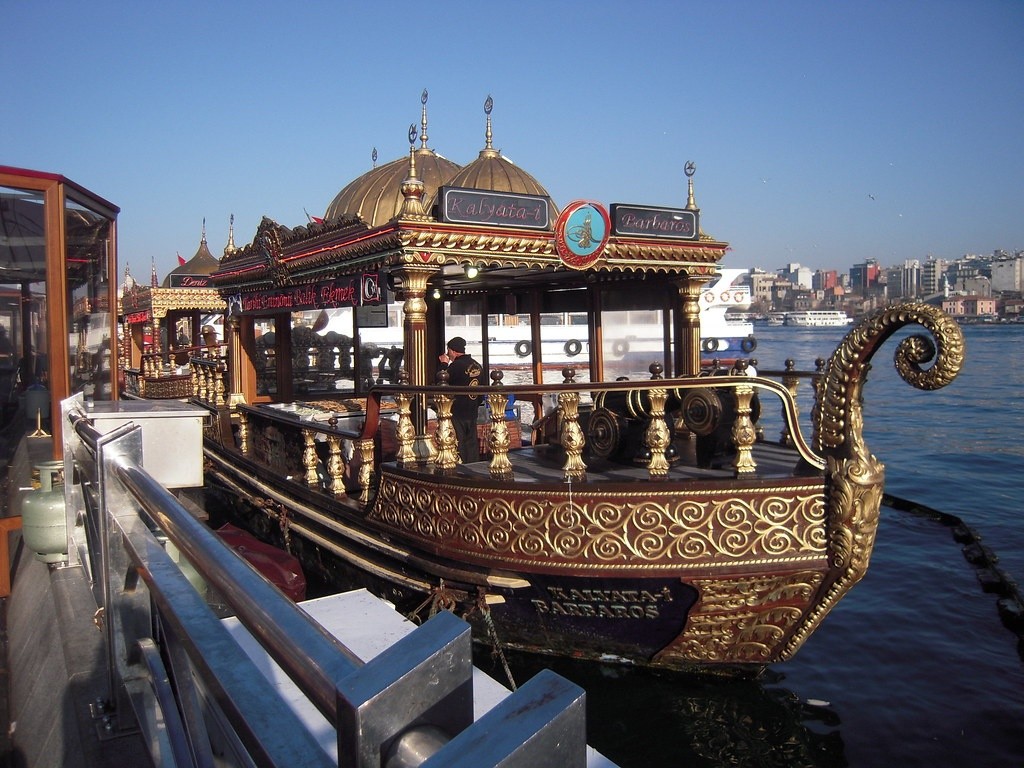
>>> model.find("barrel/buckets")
[24,383,50,419]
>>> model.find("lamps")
[26,376,53,439]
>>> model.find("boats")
[68,90,966,682]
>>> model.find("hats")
[447,337,466,353]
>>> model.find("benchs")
[380,404,522,458]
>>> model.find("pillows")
[485,394,516,419]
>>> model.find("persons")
[179,333,189,344]
[0,326,15,353]
[438,337,486,463]
